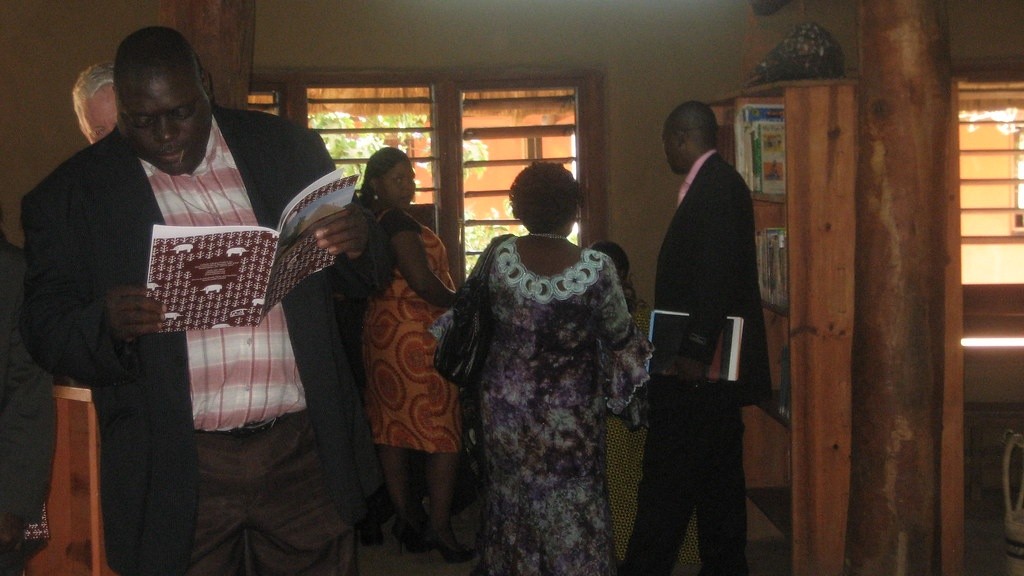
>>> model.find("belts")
[193,416,283,438]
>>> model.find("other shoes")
[360,521,383,547]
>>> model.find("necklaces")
[528,233,567,239]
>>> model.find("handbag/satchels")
[434,234,514,383]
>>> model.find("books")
[648,104,789,381]
[144,167,359,333]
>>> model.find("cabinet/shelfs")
[709,80,857,576]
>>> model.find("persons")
[361,147,475,563]
[20,28,773,576]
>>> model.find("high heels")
[391,518,429,554]
[424,526,474,564]
